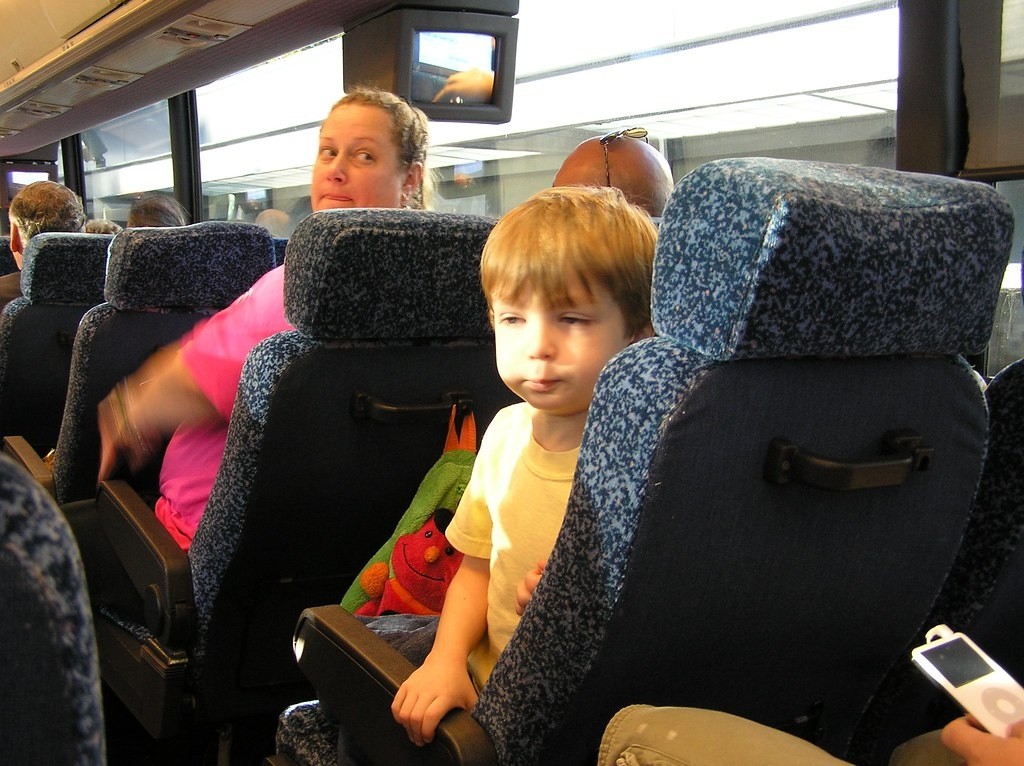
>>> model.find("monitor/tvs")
[342,8,519,125]
[0,162,58,209]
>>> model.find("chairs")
[0,157,1024,766]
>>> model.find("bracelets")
[109,387,154,458]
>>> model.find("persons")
[128,195,186,227]
[598,704,1024,766]
[0,181,87,314]
[360,183,659,745]
[99,92,429,552]
[552,125,674,216]
[256,208,289,237]
[85,219,122,234]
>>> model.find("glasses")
[600,127,648,188]
